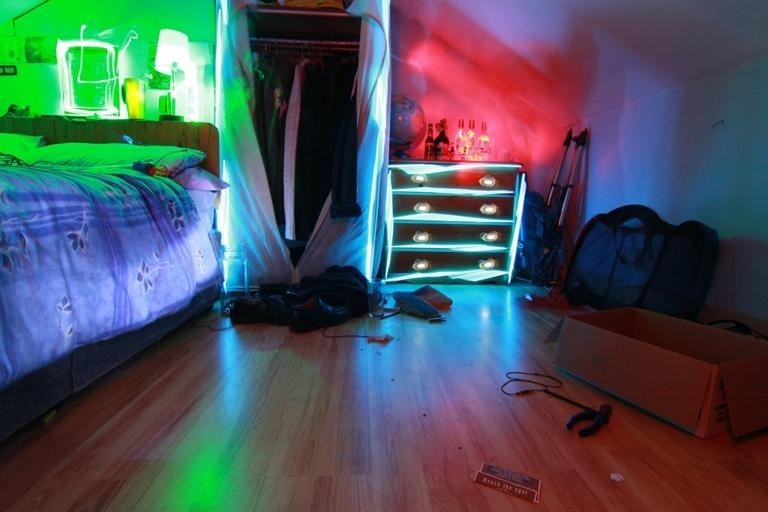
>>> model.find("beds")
[0,112,221,444]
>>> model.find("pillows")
[21,140,231,193]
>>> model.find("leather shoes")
[289,297,350,332]
[229,295,287,325]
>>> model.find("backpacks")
[516,191,557,283]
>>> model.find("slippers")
[393,292,440,318]
[413,286,453,307]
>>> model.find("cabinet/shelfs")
[387,163,520,281]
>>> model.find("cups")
[124,77,145,121]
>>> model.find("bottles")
[423,121,490,161]
[219,244,247,299]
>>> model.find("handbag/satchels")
[563,205,719,319]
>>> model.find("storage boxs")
[543,306,767,440]
